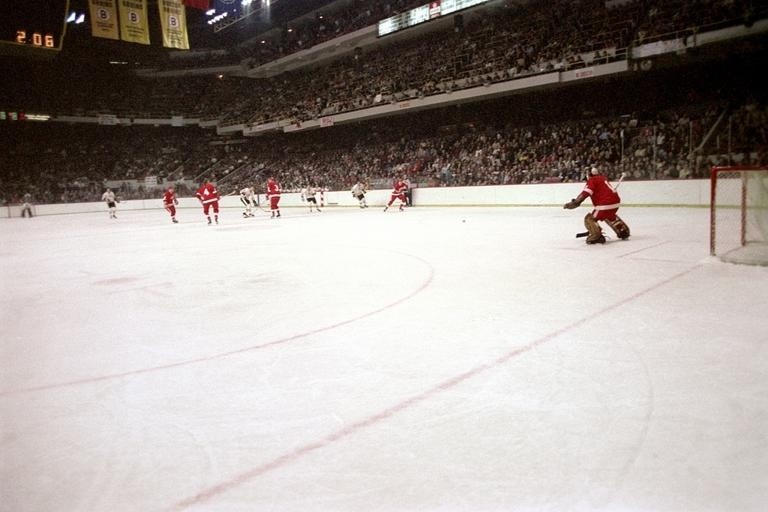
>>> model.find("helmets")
[584,166,599,180]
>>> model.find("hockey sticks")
[577,172,627,237]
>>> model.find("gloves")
[564,198,580,210]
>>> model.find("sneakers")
[586,232,630,244]
